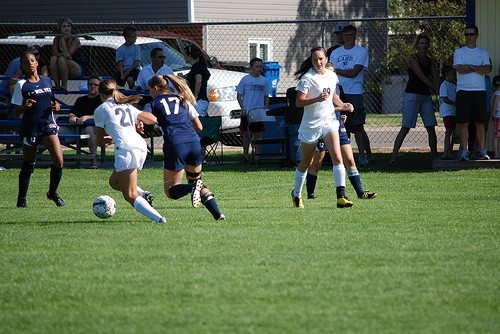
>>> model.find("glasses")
[464,33,477,36]
[88,83,99,86]
[157,56,165,59]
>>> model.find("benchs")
[0,74,162,162]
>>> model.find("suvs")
[0,30,250,144]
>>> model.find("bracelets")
[242,107,245,109]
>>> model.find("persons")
[307,61,377,200]
[453,24,492,160]
[0,45,49,120]
[326,25,374,166]
[290,46,354,208]
[94,80,167,224]
[11,52,66,209]
[439,66,457,160]
[235,58,270,164]
[50,17,83,89]
[133,47,177,92]
[138,74,227,222]
[116,26,143,91]
[388,35,438,166]
[183,44,211,164]
[471,58,500,159]
[68,75,104,168]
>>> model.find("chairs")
[197,116,224,171]
[247,107,286,166]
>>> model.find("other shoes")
[129,86,142,90]
[202,160,207,165]
[243,158,256,164]
[118,80,125,87]
[357,154,374,166]
[51,86,68,91]
[323,156,331,165]
[89,160,98,168]
[390,157,400,167]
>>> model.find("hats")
[250,58,262,67]
[333,24,344,33]
[492,76,500,86]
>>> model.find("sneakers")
[291,190,304,209]
[17,203,27,208]
[308,193,314,200]
[143,191,153,207]
[156,217,166,224]
[337,196,353,208]
[440,150,500,161]
[217,214,226,224]
[191,179,203,209]
[358,190,377,199]
[47,191,66,207]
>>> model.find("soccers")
[93,195,118,219]
